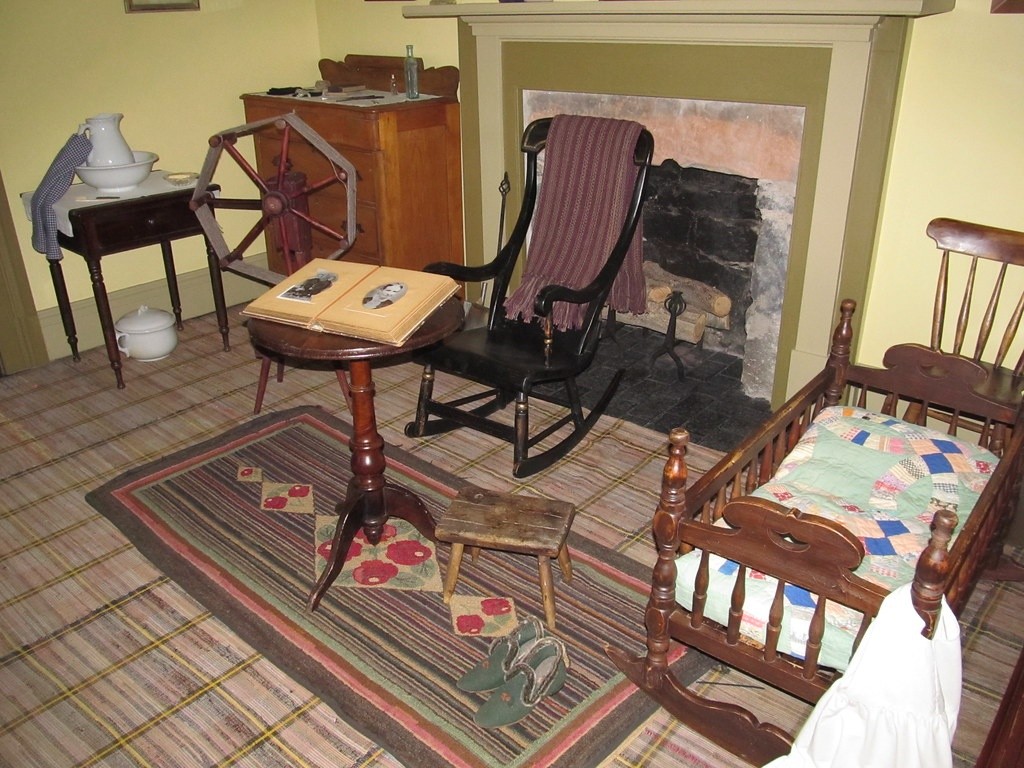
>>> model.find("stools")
[432,486,574,631]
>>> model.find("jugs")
[76,111,136,168]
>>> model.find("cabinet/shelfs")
[240,53,464,302]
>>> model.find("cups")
[116,306,179,363]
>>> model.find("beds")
[601,299,1024,764]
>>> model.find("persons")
[362,282,404,310]
[287,273,336,298]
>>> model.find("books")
[239,257,461,348]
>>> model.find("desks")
[17,168,231,389]
[245,294,469,615]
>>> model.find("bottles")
[404,44,421,100]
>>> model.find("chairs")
[398,113,655,481]
[905,216,1024,452]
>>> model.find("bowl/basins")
[70,150,159,191]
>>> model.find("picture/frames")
[124,0,204,13]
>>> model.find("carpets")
[83,405,723,768]
